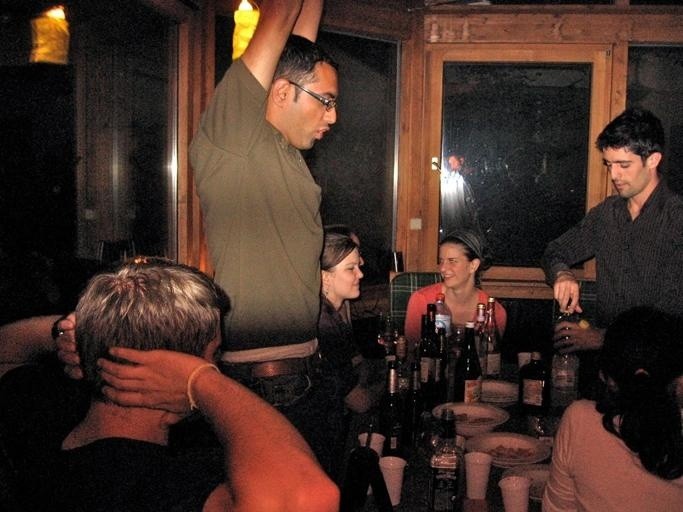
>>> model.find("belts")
[251,351,323,378]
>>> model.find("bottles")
[378,292,500,452]
[517,308,579,415]
[427,405,465,512]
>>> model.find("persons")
[318,232,386,414]
[540,305,683,510]
[0,256,340,511]
[444,151,484,193]
[186,0,339,491]
[343,228,366,318]
[402,229,508,345]
[536,107,682,354]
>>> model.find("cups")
[357,432,386,455]
[463,451,493,500]
[497,477,533,511]
[378,456,407,506]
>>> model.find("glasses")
[288,77,338,110]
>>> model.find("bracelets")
[52,312,68,345]
[186,363,220,410]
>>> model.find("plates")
[499,463,551,501]
[431,377,551,466]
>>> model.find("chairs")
[391,270,443,323]
